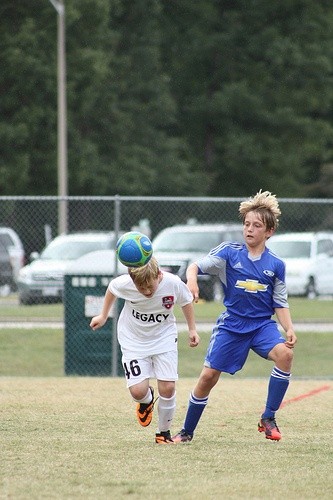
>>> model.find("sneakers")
[171,429,193,443]
[137,386,159,427]
[155,430,175,445]
[257,417,282,440]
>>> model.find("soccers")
[115,230,153,267]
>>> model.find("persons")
[88,256,199,445]
[170,188,297,445]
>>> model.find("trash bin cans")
[64,273,127,378]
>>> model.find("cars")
[0,227,26,292]
[152,224,244,303]
[265,232,333,298]
[16,230,125,305]
